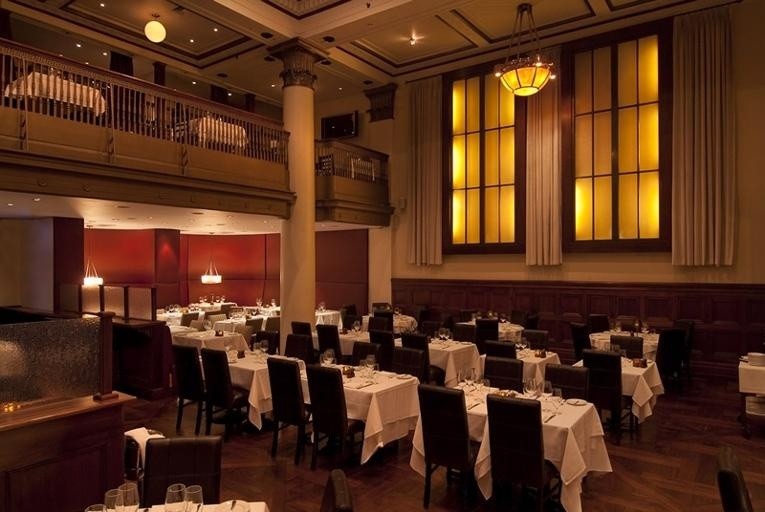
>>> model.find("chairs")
[564,313,584,339]
[246,318,264,333]
[417,384,481,512]
[483,356,524,395]
[291,320,312,334]
[544,363,590,402]
[305,363,366,476]
[265,316,281,333]
[284,333,314,363]
[350,342,383,371]
[483,340,517,359]
[189,320,203,331]
[401,332,444,386]
[171,343,207,436]
[569,321,593,362]
[342,303,362,330]
[372,302,392,316]
[391,346,426,384]
[476,319,499,355]
[235,323,253,345]
[715,444,754,512]
[655,320,698,397]
[368,316,394,333]
[267,357,313,466]
[244,306,260,317]
[374,309,393,317]
[180,312,200,327]
[200,347,260,444]
[486,394,562,512]
[228,306,243,318]
[459,308,478,322]
[316,324,342,363]
[520,329,550,350]
[213,321,234,332]
[319,469,354,512]
[589,313,610,333]
[142,435,223,509]
[526,315,538,330]
[583,348,634,447]
[510,309,524,325]
[609,334,643,360]
[369,329,395,359]
[207,314,227,330]
[443,314,457,333]
[254,330,280,356]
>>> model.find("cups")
[183,485,203,512]
[83,503,105,512]
[102,489,123,512]
[114,483,139,512]
[164,484,185,512]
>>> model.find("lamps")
[144,13,167,43]
[81,225,104,286]
[493,2,560,97]
[201,232,222,284]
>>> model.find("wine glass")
[438,328,444,343]
[202,319,212,336]
[518,337,526,351]
[259,340,268,357]
[479,379,490,402]
[550,388,563,415]
[447,332,453,346]
[358,360,366,378]
[443,329,450,344]
[203,296,207,303]
[499,313,506,328]
[319,354,326,367]
[523,378,535,398]
[168,304,173,313]
[457,368,466,391]
[464,368,474,392]
[513,337,522,350]
[323,352,331,367]
[215,296,219,303]
[198,296,203,304]
[539,381,553,403]
[352,320,359,335]
[220,297,224,303]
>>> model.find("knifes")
[467,403,480,410]
[543,415,556,423]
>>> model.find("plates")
[747,353,764,366]
[566,399,586,406]
[396,374,411,379]
